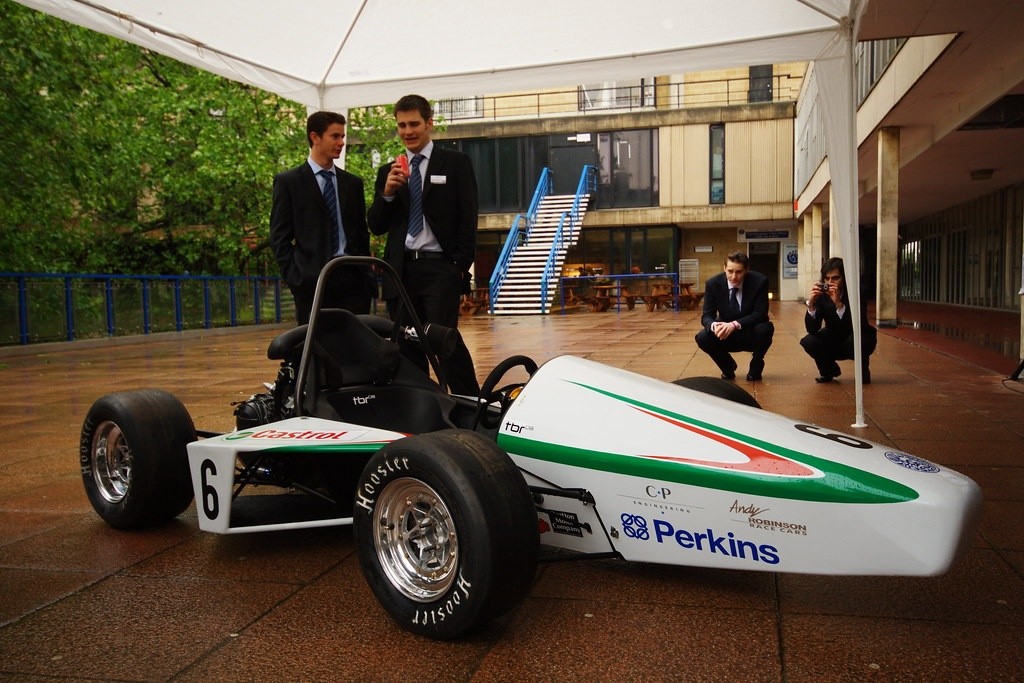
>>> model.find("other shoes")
[862,367,871,384]
[815,363,841,383]
[747,361,764,380]
[721,360,738,379]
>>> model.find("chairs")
[294,256,458,436]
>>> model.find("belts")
[406,249,449,260]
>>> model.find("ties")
[408,155,426,237]
[318,170,340,256]
[728,288,740,320]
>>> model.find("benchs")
[585,293,705,312]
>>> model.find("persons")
[270,111,380,325]
[366,94,480,396]
[799,257,877,384]
[695,250,775,381]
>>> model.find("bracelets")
[732,321,737,331]
[805,299,816,307]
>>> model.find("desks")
[594,286,629,297]
[653,282,693,295]
